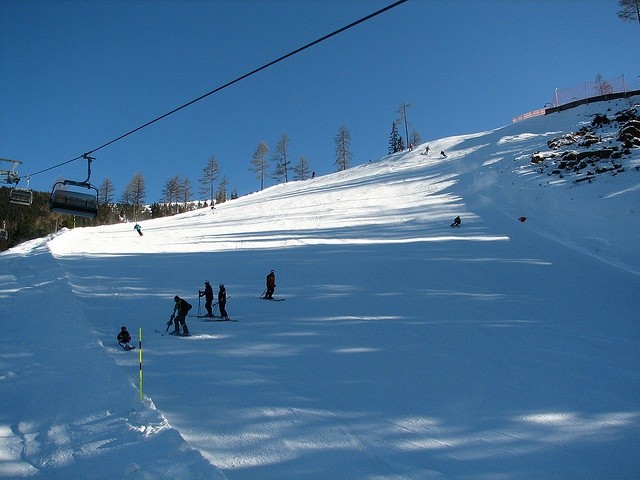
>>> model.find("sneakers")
[223,317,229,320]
[182,331,189,334]
[125,345,130,350]
[171,329,180,334]
[219,316,224,320]
[206,312,212,316]
[132,345,136,349]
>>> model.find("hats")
[173,294,179,301]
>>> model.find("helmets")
[219,283,224,288]
[270,270,275,273]
[204,279,209,284]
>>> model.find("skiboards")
[155,330,192,336]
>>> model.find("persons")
[263,270,276,299]
[173,295,192,335]
[199,280,213,317]
[117,326,136,351]
[450,216,461,226]
[218,283,231,321]
[133,222,144,237]
